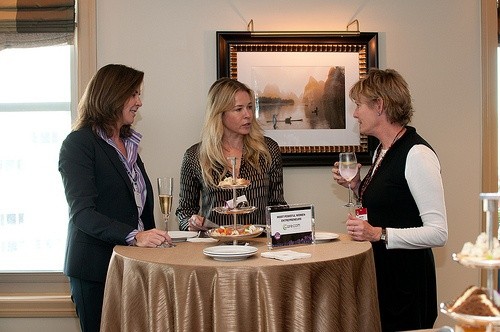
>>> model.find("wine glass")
[339,152,360,208]
[157,177,177,248]
[198,188,217,237]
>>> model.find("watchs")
[381,227,386,242]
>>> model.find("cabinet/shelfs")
[440,190,500,332]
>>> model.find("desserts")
[448,232,500,332]
[212,176,256,235]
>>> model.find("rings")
[352,232,354,235]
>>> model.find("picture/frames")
[216,30,379,168]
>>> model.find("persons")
[58,64,172,332]
[332,69,448,332]
[175,77,288,238]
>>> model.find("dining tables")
[98,230,380,332]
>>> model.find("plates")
[312,232,339,242]
[168,230,198,242]
[203,245,259,262]
